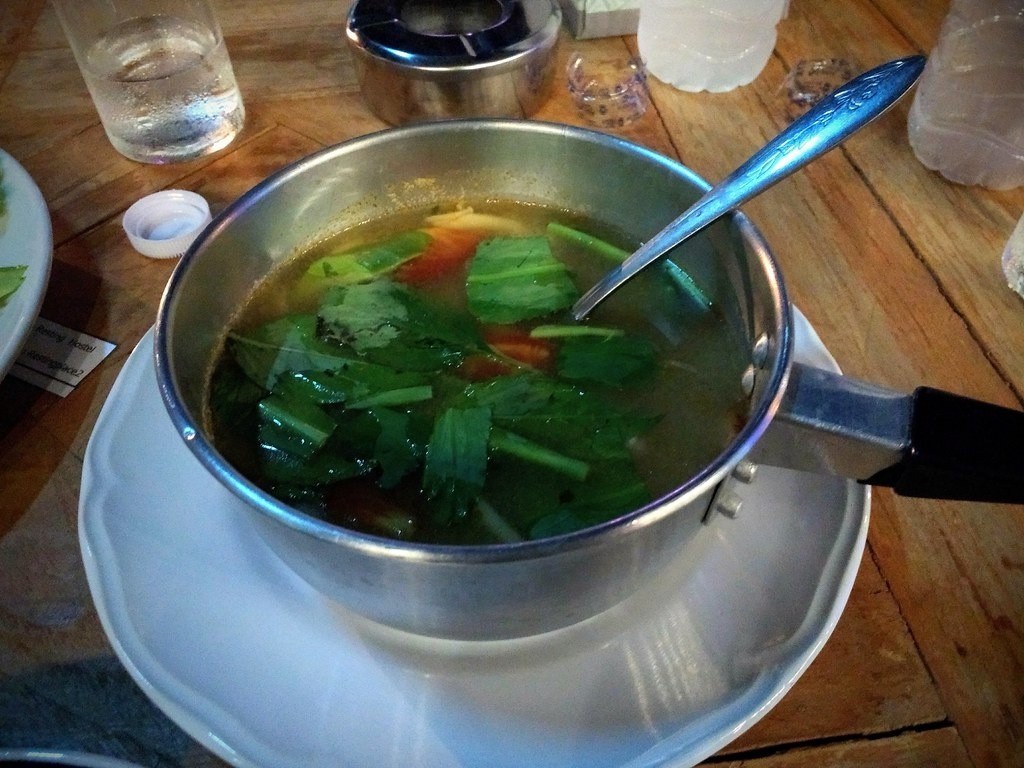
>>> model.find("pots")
[153,120,1024,642]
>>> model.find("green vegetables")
[208,219,716,549]
[0,265,28,299]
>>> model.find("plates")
[76,301,872,768]
[0,150,54,384]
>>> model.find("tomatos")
[393,226,487,283]
[471,329,555,379]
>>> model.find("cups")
[51,0,246,164]
[637,0,787,92]
[908,0,1024,190]
[1001,211,1024,299]
